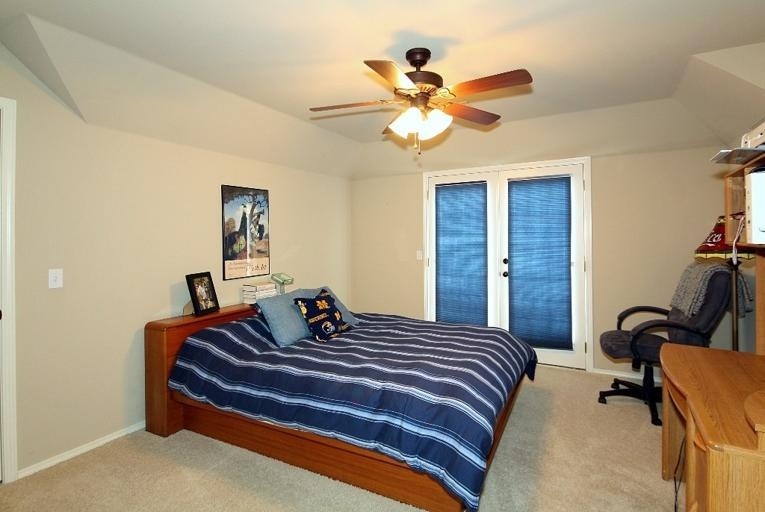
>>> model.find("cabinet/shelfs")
[660,155,765,356]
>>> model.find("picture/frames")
[222,185,270,280]
[185,271,220,315]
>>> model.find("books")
[241,281,280,305]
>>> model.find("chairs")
[598,261,731,423]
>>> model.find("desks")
[660,343,765,512]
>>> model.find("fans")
[310,49,530,125]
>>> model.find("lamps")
[696,216,753,351]
[271,273,294,293]
[388,111,454,156]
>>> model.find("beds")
[144,304,538,512]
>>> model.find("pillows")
[258,286,357,351]
[294,288,351,342]
